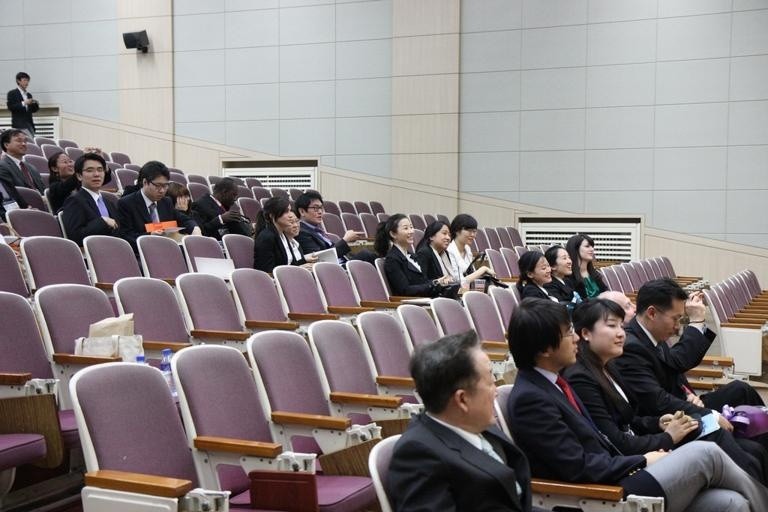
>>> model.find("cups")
[460,277,471,291]
[474,278,486,293]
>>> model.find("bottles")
[159,348,178,398]
[136,355,149,366]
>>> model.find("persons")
[390,329,532,512]
[49,146,253,260]
[507,296,768,511]
[254,190,377,273]
[374,212,613,307]
[560,298,768,486]
[5,72,40,139]
[608,276,767,483]
[595,286,768,413]
[0,129,47,210]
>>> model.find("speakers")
[123,31,149,49]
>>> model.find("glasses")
[304,206,324,211]
[147,179,171,190]
[79,166,106,174]
[649,302,683,326]
[556,322,575,338]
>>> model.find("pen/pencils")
[663,418,698,425]
